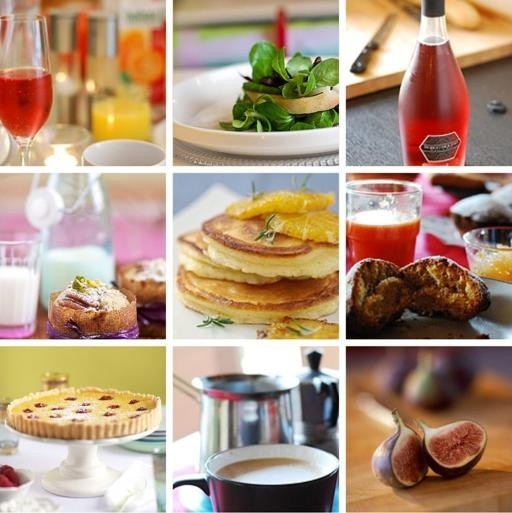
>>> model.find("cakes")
[6,387,162,440]
[49,275,137,335]
[115,257,166,305]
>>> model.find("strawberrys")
[1,465,20,488]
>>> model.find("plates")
[173,183,339,340]
[346,276,512,340]
[173,59,339,158]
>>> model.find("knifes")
[350,14,396,74]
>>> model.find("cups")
[28,173,115,315]
[297,351,340,427]
[172,442,339,512]
[0,231,43,339]
[36,10,166,166]
[463,226,512,284]
[346,178,423,282]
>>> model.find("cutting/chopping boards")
[346,0,512,99]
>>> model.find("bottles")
[398,0,470,166]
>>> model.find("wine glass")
[1,13,54,166]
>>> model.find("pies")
[175,214,338,325]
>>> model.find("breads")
[346,258,418,335]
[448,180,512,242]
[398,256,492,321]
